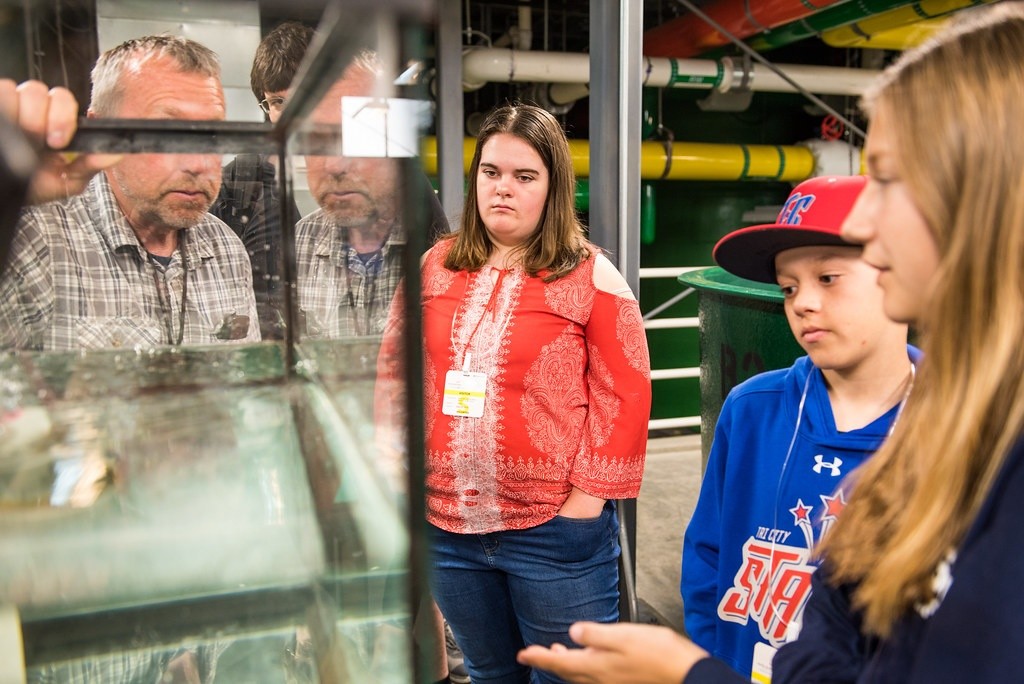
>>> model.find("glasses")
[259,96,291,114]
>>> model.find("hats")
[710,176,872,285]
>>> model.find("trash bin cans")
[677,266,807,478]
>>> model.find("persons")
[210,19,330,683]
[681,177,926,683]
[373,105,652,683]
[0,35,260,684]
[516,9,1024,684]
[296,53,411,336]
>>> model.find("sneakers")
[443,618,471,684]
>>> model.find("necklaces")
[111,189,187,346]
[342,224,393,336]
